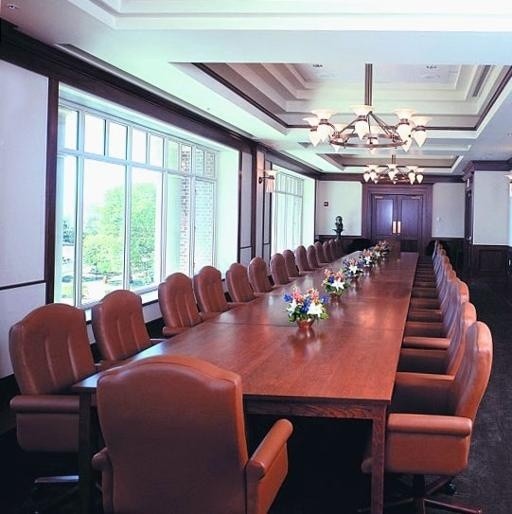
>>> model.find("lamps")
[361,156,427,187]
[302,62,429,155]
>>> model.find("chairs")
[3,233,495,514]
